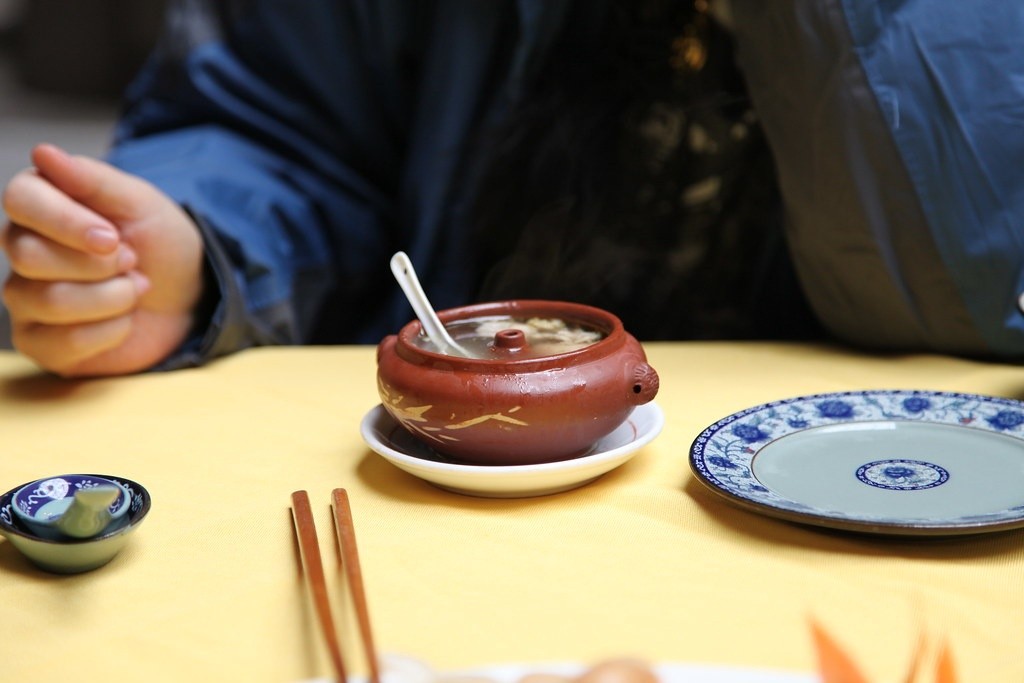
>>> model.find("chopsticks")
[292,489,380,683]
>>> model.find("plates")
[690,390,1024,535]
[360,400,664,499]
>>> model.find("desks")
[1,342,1024,683]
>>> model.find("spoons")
[14,475,131,541]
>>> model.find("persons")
[0,1,1022,377]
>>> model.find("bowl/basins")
[378,301,659,465]
[0,475,151,572]
